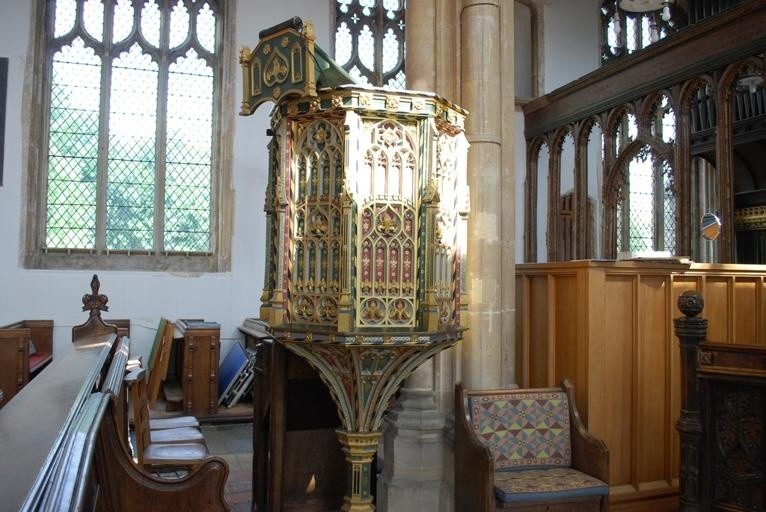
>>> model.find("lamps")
[662,1,671,21]
[614,0,621,33]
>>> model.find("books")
[216,340,256,409]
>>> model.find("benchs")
[0,319,268,414]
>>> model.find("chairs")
[456,381,610,511]
[126,356,209,512]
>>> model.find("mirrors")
[700,213,722,241]
